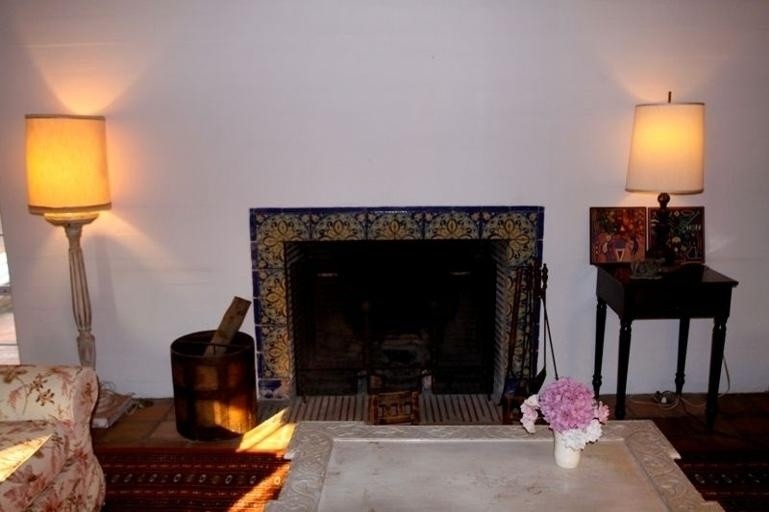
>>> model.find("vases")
[554,432,580,470]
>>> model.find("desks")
[593,264,738,420]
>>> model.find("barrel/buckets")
[170,330,259,441]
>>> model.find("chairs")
[0,365,106,512]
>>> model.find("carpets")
[95,444,769,512]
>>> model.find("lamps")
[625,91,705,264]
[25,113,133,429]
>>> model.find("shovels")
[531,263,548,395]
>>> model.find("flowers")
[520,377,611,451]
